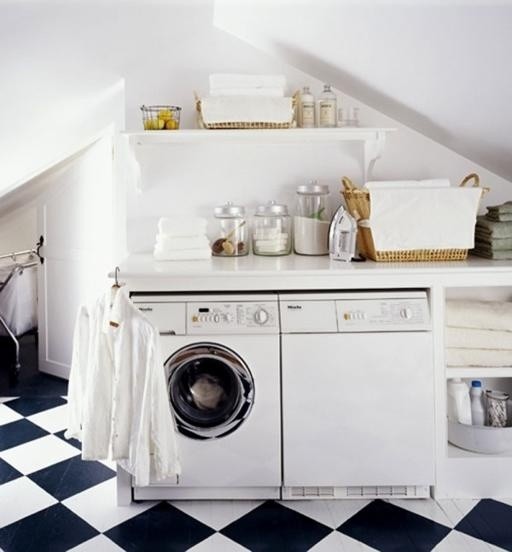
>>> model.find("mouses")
[109,266,121,328]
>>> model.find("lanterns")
[157,110,174,120]
[150,119,165,130]
[166,119,179,130]
[143,119,151,130]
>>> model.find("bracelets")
[446,414,511,457]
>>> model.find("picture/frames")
[108,253,512,507]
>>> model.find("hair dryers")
[130,295,282,502]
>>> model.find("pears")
[446,379,486,426]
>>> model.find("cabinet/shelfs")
[348,106,361,128]
[293,184,337,255]
[445,374,472,428]
[210,204,249,257]
[337,108,348,127]
[318,83,340,129]
[297,85,316,129]
[465,379,487,426]
[252,201,292,256]
